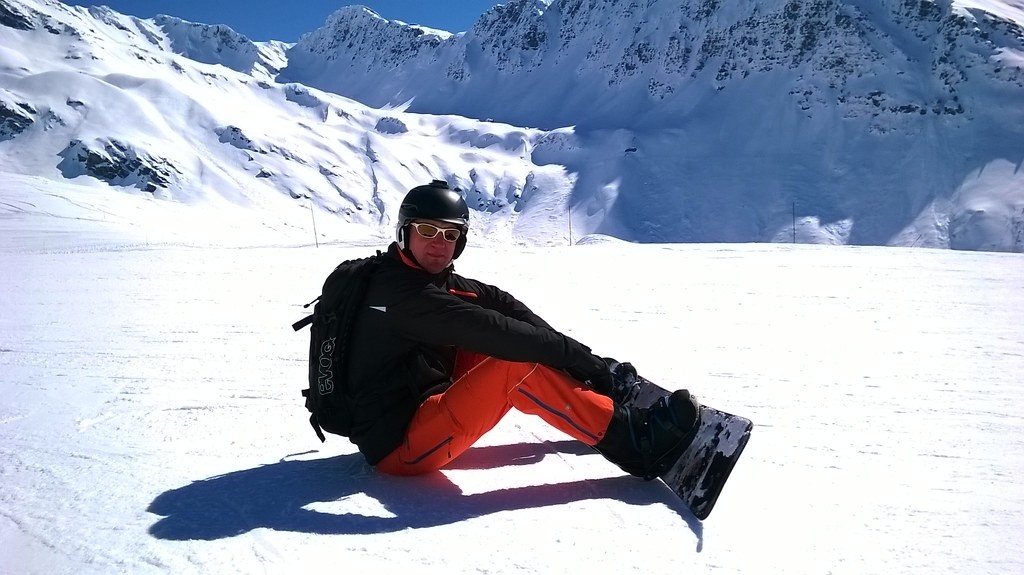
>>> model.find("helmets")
[395,179,469,260]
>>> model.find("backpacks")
[291,257,413,443]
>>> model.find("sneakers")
[595,389,693,477]
[603,361,636,407]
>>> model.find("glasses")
[411,222,460,243]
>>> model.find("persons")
[350,180,699,478]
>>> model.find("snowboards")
[599,355,754,522]
